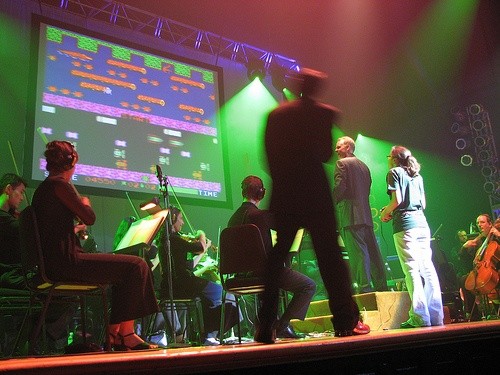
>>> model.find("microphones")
[157,165,163,188]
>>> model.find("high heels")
[104,332,159,351]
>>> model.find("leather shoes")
[400,319,443,328]
[278,325,305,338]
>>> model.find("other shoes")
[205,337,220,345]
[352,320,370,334]
[65,341,103,352]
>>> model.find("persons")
[228,175,316,344]
[254,68,360,345]
[332,136,386,293]
[31,140,159,352]
[449,229,475,275]
[457,214,500,322]
[379,146,444,328]
[159,206,244,346]
[0,173,28,262]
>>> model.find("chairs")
[144,264,204,347]
[0,206,111,359]
[218,224,288,343]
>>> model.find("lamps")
[247,61,264,82]
[448,103,500,194]
[368,194,381,234]
[272,73,287,92]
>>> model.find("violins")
[75,216,88,241]
[180,233,217,255]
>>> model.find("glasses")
[387,156,397,160]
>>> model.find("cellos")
[464,218,500,323]
